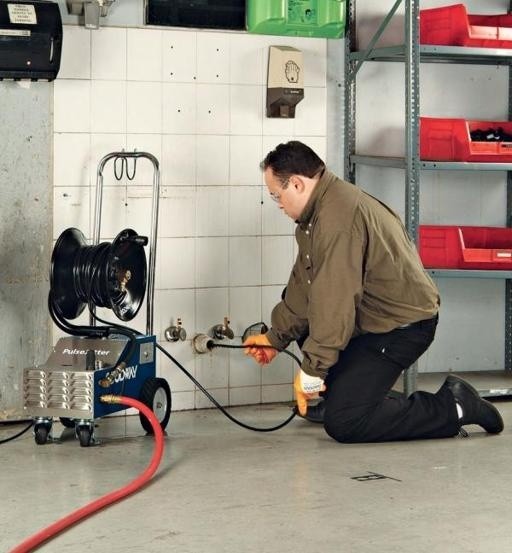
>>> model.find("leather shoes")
[444,373,503,434]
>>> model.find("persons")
[241,140,505,444]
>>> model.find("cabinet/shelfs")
[345,0,511,417]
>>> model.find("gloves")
[292,369,326,416]
[244,334,279,365]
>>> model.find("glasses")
[270,178,289,202]
